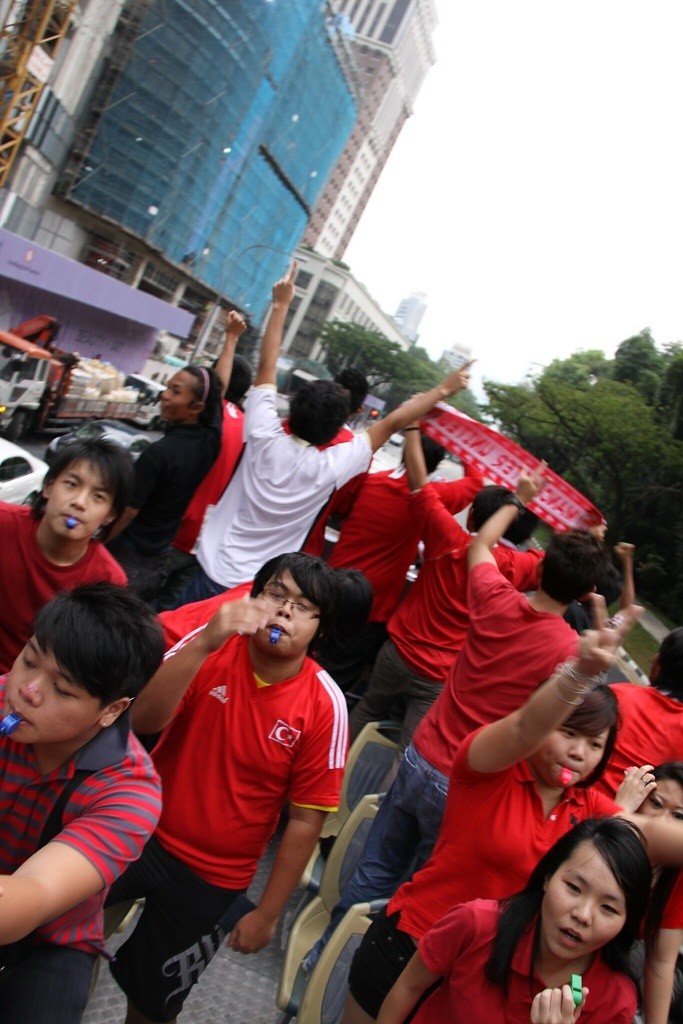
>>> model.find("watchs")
[503,493,525,514]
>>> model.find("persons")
[377,813,654,1024]
[1,259,552,1024]
[619,759,683,1024]
[299,460,607,968]
[340,606,682,1023]
[564,519,636,638]
[592,626,683,805]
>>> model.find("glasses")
[260,588,320,622]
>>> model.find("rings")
[641,778,648,784]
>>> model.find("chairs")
[297,898,393,1024]
[88,898,144,1001]
[280,720,405,955]
[276,792,388,1024]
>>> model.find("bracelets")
[401,427,419,432]
[550,655,601,706]
